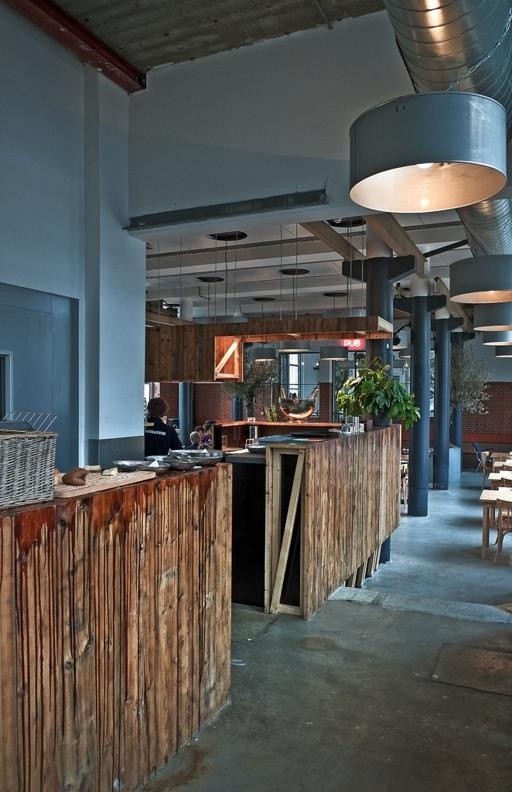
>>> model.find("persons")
[186,430,203,449]
[203,419,215,434]
[144,398,181,456]
[195,426,212,449]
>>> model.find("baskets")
[1,430,57,510]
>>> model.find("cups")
[342,416,365,436]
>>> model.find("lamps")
[275,222,348,360]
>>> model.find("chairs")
[471,442,512,565]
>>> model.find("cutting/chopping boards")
[54,472,156,499]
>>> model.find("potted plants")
[336,356,420,431]
[222,353,280,420]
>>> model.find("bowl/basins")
[144,455,199,471]
[112,460,170,474]
[170,449,223,466]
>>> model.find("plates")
[246,433,293,453]
